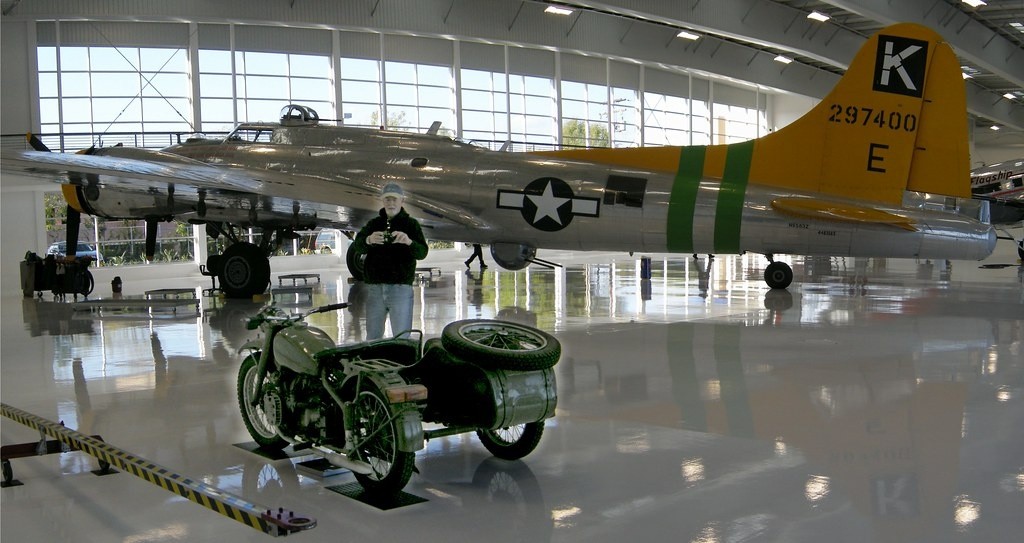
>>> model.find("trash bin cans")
[20,260,89,299]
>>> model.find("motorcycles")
[236,282,561,495]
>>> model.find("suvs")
[315,228,357,255]
[47,241,103,263]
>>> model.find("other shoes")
[693,254,698,259]
[708,254,715,259]
[464,261,471,269]
[480,264,488,268]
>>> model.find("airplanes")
[0,22,1024,304]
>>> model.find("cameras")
[380,232,396,243]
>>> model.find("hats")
[381,183,404,198]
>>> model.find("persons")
[353,183,429,342]
[464,242,489,269]
[693,253,714,260]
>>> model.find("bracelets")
[368,234,375,248]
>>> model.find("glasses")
[382,200,401,206]
